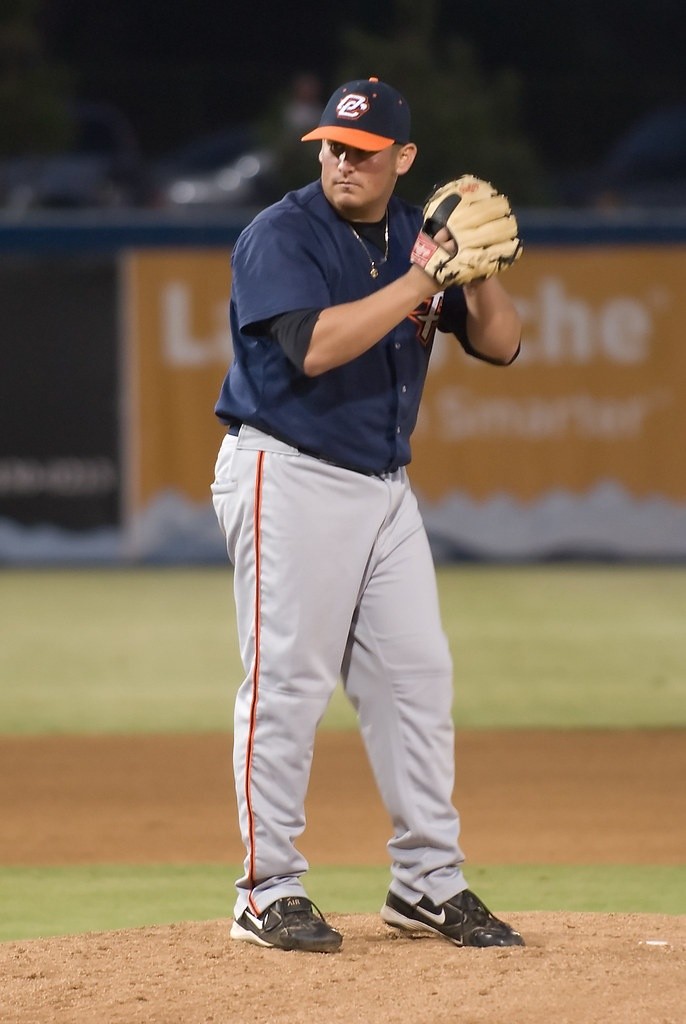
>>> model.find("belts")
[228,423,243,437]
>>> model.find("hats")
[301,77,411,151]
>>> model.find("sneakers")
[380,889,525,948]
[229,896,343,952]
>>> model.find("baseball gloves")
[410,174,523,285]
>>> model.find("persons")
[209,76,526,953]
